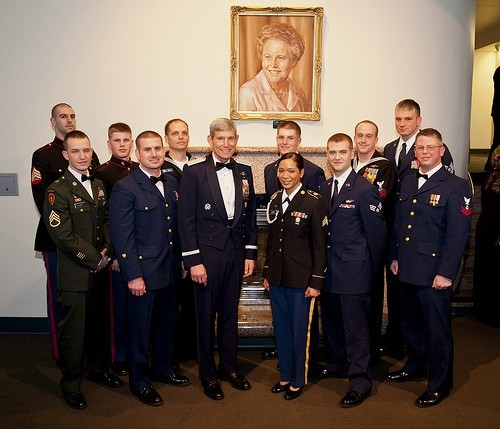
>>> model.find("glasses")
[415,144,442,151]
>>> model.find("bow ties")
[215,161,234,170]
[150,173,167,183]
[415,173,428,181]
[81,174,96,183]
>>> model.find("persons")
[43,131,122,410]
[108,131,190,406]
[263,152,328,400]
[387,129,471,407]
[261,99,456,373]
[238,22,308,112]
[32,103,199,377]
[178,117,259,400]
[315,133,384,408]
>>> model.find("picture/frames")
[229,6,324,121]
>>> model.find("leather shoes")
[261,348,278,359]
[384,369,425,382]
[284,385,304,400]
[218,369,249,389]
[375,341,390,357]
[202,376,224,400]
[114,365,128,376]
[271,380,291,393]
[130,383,164,406]
[310,366,334,378]
[88,369,123,388]
[151,372,190,387]
[340,391,369,407]
[414,387,446,407]
[62,391,87,410]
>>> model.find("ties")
[331,180,338,211]
[398,143,407,166]
[282,197,289,214]
